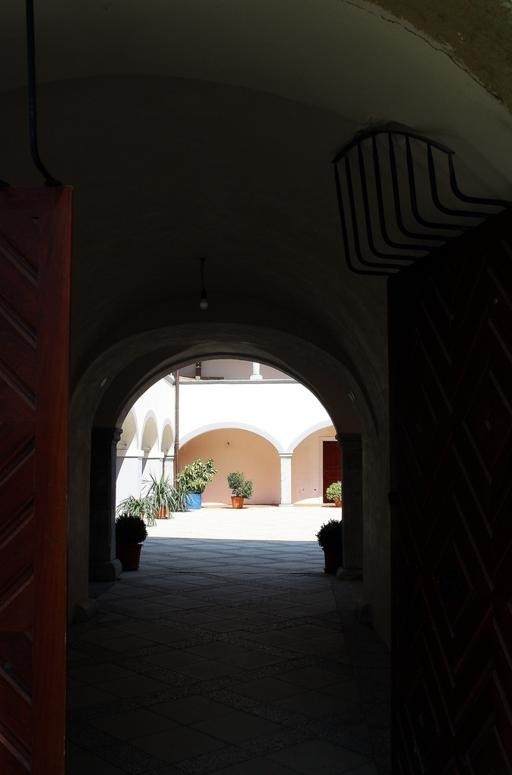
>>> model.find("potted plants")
[117,512,147,572]
[316,518,342,571]
[141,472,178,519]
[227,471,255,509]
[326,480,342,507]
[176,457,216,509]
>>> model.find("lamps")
[198,253,212,312]
